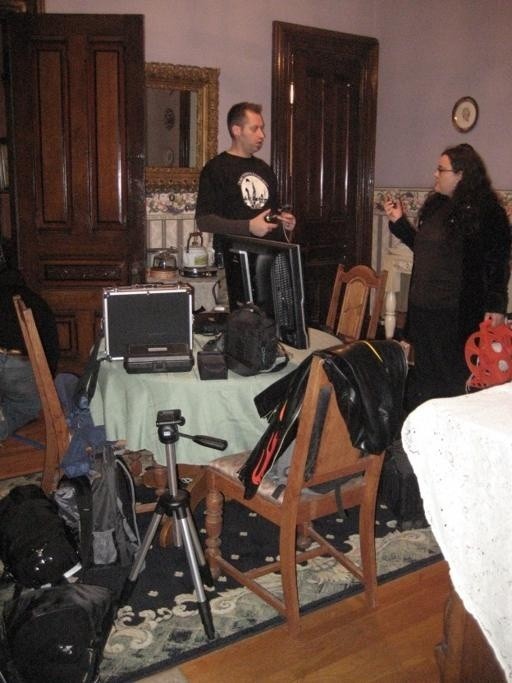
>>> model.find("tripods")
[119,410,227,640]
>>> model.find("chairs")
[13,294,172,525]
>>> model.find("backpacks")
[49,445,146,601]
[0,484,81,588]
[0,585,112,683]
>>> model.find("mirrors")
[144,62,219,195]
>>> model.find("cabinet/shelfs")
[2,12,145,372]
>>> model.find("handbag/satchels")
[224,303,290,377]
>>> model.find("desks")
[91,327,344,550]
[381,253,414,339]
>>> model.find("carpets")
[0,451,443,683]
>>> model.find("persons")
[381,143,512,412]
[193,100,298,276]
[0,264,63,443]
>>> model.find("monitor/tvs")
[217,232,310,358]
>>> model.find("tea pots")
[180,231,209,267]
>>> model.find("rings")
[287,222,289,227]
